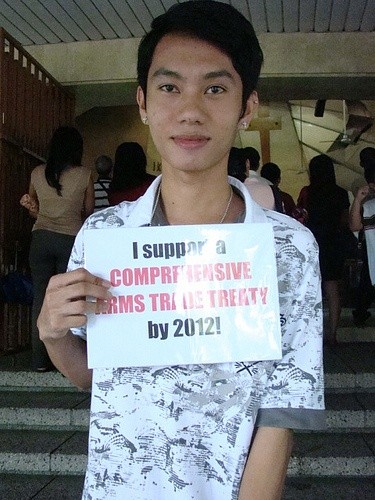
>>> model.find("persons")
[258,162,299,221]
[109,141,153,207]
[28,126,96,371]
[91,155,112,214]
[351,147,375,198]
[228,146,275,214]
[242,146,274,187]
[35,1,328,500]
[346,156,375,325]
[295,153,355,356]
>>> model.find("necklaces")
[150,183,234,226]
